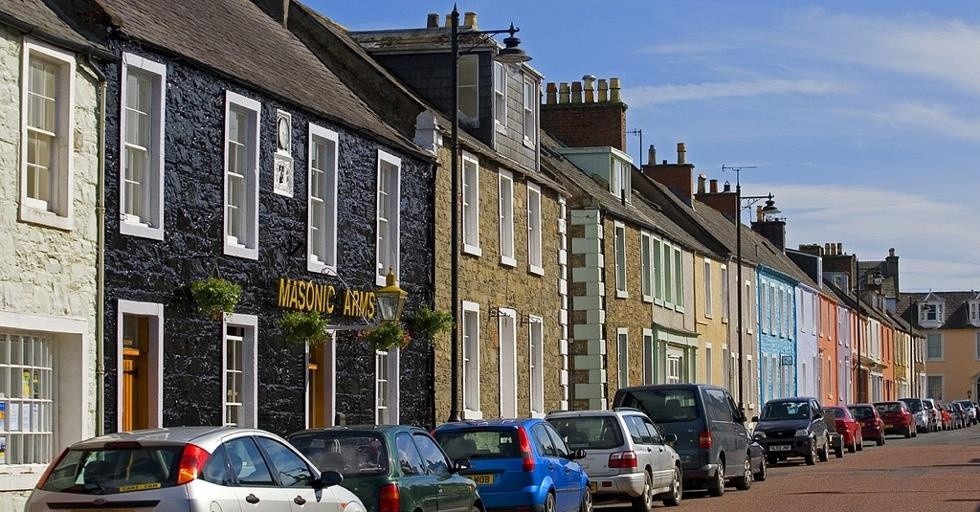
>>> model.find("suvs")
[823,398,978,459]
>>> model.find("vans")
[612,384,753,496]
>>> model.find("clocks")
[278,117,289,149]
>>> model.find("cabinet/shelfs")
[0,359,55,465]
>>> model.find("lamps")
[321,265,408,328]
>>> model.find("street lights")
[447,2,533,421]
[910,295,931,398]
[736,180,781,415]
[856,259,886,404]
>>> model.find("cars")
[284,425,486,512]
[752,396,831,466]
[746,431,768,482]
[546,410,684,510]
[432,419,595,512]
[24,425,366,512]
